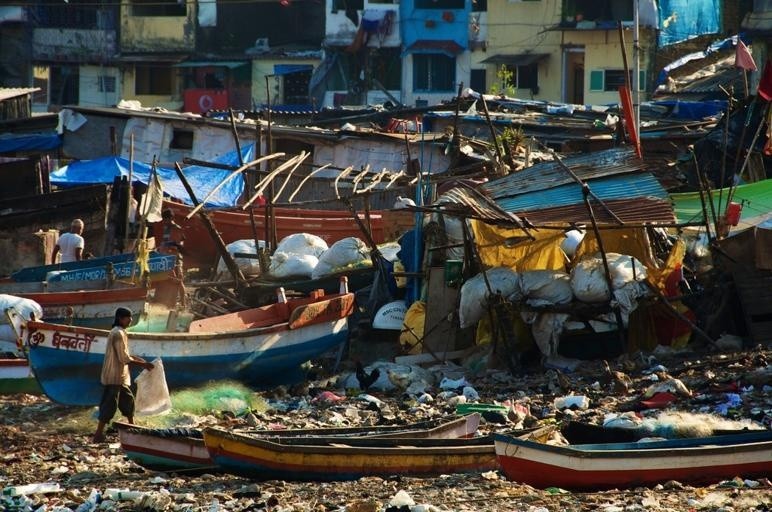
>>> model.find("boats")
[201,422,558,483]
[0,248,355,409]
[488,429,772,492]
[554,409,771,452]
[117,402,509,476]
[144,195,439,254]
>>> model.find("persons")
[52,218,85,265]
[159,208,186,307]
[93,306,153,444]
